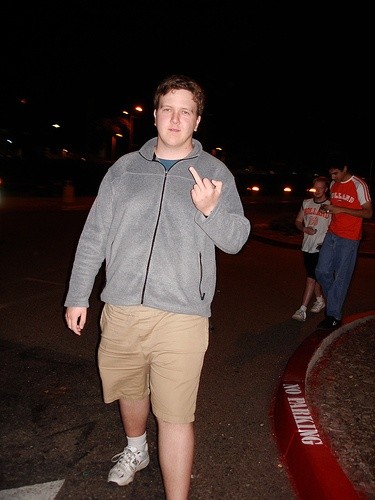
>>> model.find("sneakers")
[310,299,326,313]
[107,439,150,487]
[291,309,307,322]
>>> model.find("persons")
[290,176,334,322]
[315,159,372,329]
[64,76,251,500]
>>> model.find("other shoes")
[316,316,340,331]
